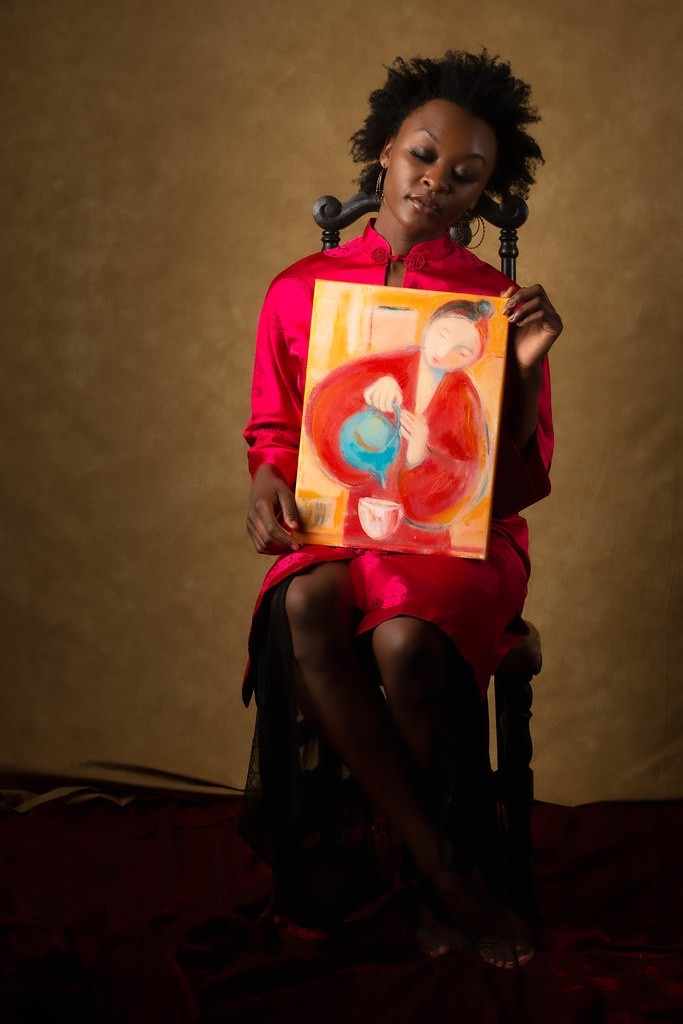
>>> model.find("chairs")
[254,182,544,954]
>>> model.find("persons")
[241,39,564,966]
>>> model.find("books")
[290,279,509,559]
